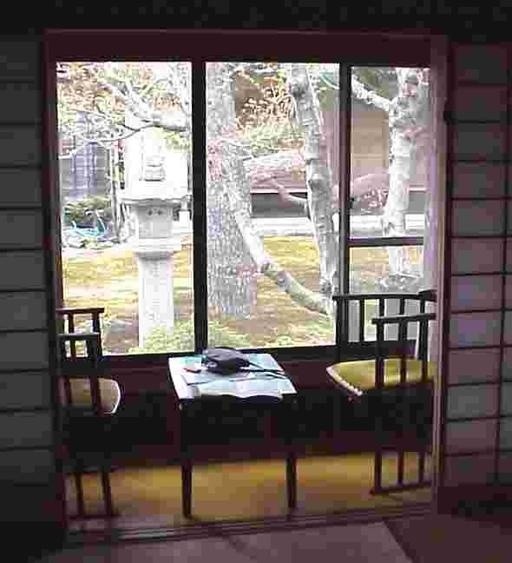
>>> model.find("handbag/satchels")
[201,348,288,378]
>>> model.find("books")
[181,370,282,403]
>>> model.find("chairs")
[56,306,120,519]
[323,290,436,494]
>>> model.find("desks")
[167,352,298,515]
